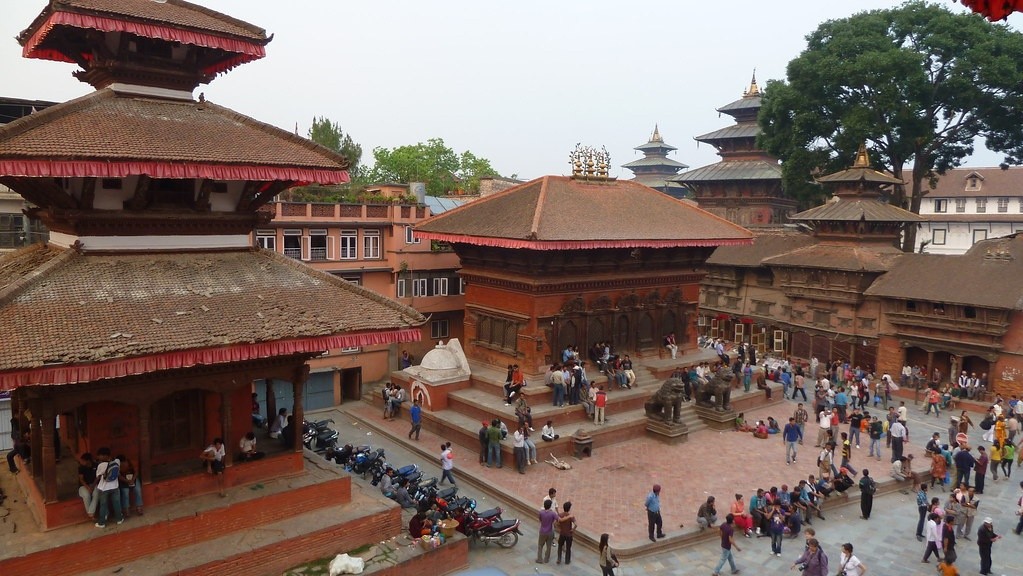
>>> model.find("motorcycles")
[300,417,524,548]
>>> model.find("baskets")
[440,518,459,537]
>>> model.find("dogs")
[545,452,572,470]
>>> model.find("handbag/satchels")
[606,554,619,569]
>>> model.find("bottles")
[418,532,446,551]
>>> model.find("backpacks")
[102,459,120,482]
[864,476,876,495]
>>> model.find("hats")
[983,517,992,524]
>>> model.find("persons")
[663,330,680,362]
[399,350,412,370]
[697,469,876,576]
[438,441,457,488]
[645,485,666,542]
[500,341,636,432]
[199,437,227,498]
[535,488,577,564]
[479,418,559,475]
[77,446,145,528]
[599,534,619,576]
[7,409,42,479]
[380,467,457,539]
[382,381,405,420]
[407,399,423,440]
[237,431,266,463]
[917,480,1023,576]
[250,393,260,414]
[270,408,295,450]
[326,444,354,472]
[672,335,1023,496]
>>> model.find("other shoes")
[94,522,105,528]
[799,514,825,526]
[135,507,144,516]
[930,486,933,489]
[786,456,796,465]
[746,527,760,537]
[116,518,124,523]
[608,383,631,391]
[866,453,881,461]
[922,559,929,563]
[856,445,860,449]
[479,460,501,469]
[649,534,666,542]
[917,535,922,542]
[994,476,1010,482]
[519,458,538,474]
[769,550,781,557]
[123,508,132,518]
[940,486,945,493]
[533,557,572,565]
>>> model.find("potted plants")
[280,188,418,203]
[448,188,476,194]
